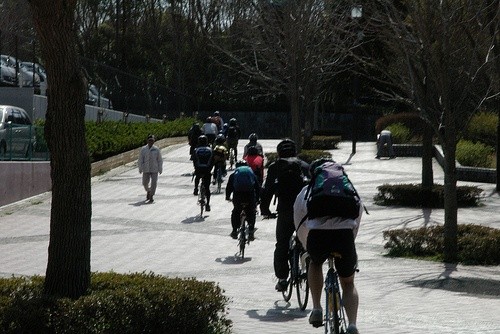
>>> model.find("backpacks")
[214,145,227,161]
[295,162,370,232]
[272,159,313,206]
[196,147,212,168]
[232,166,255,197]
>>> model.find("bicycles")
[262,213,311,311]
[188,140,234,218]
[226,197,255,261]
[291,236,349,334]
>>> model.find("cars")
[0,105,36,159]
[0,54,98,105]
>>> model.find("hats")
[147,136,155,142]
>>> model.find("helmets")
[247,146,258,155]
[235,161,245,168]
[276,139,297,153]
[192,111,237,142]
[248,133,258,140]
[308,157,336,176]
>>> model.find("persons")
[137,135,163,202]
[244,133,263,158]
[226,160,260,241]
[375,130,394,159]
[188,111,241,185]
[259,139,309,291]
[245,146,263,183]
[190,135,213,211]
[294,158,363,334]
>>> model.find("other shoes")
[230,231,238,239]
[193,188,199,195]
[213,179,216,185]
[347,326,360,334]
[205,205,210,212]
[275,280,288,291]
[226,156,229,160]
[309,310,323,325]
[147,191,154,202]
[248,233,254,241]
[190,155,194,161]
[223,172,227,177]
[234,155,237,162]
